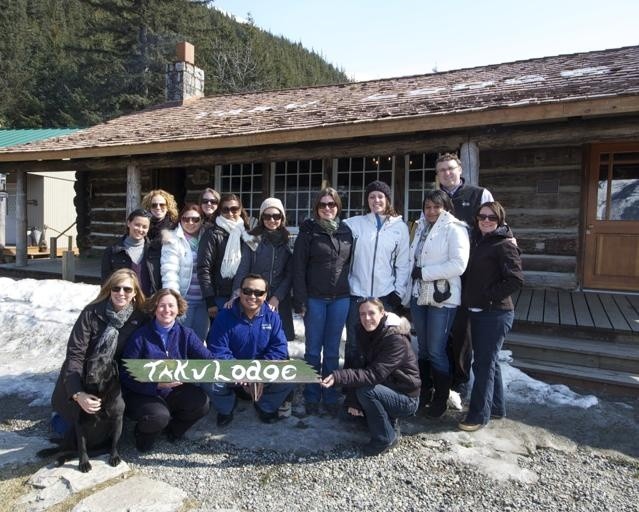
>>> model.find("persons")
[47,152,521,456]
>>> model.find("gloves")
[481,299,498,314]
[386,292,402,307]
[411,266,422,280]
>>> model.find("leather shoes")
[216,397,238,426]
[253,400,278,422]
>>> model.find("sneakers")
[134,422,155,451]
[457,415,483,431]
[325,401,340,413]
[277,400,292,419]
[447,389,464,411]
[424,401,449,419]
[305,403,320,414]
[361,436,399,456]
[489,413,505,419]
[236,384,252,401]
[166,421,176,440]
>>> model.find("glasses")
[221,206,240,213]
[181,216,201,224]
[240,286,267,297]
[151,202,167,209]
[111,285,134,294]
[202,198,217,204]
[261,213,282,221]
[317,201,337,209]
[476,213,498,222]
[436,164,460,172]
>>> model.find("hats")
[365,180,392,200]
[258,197,286,226]
